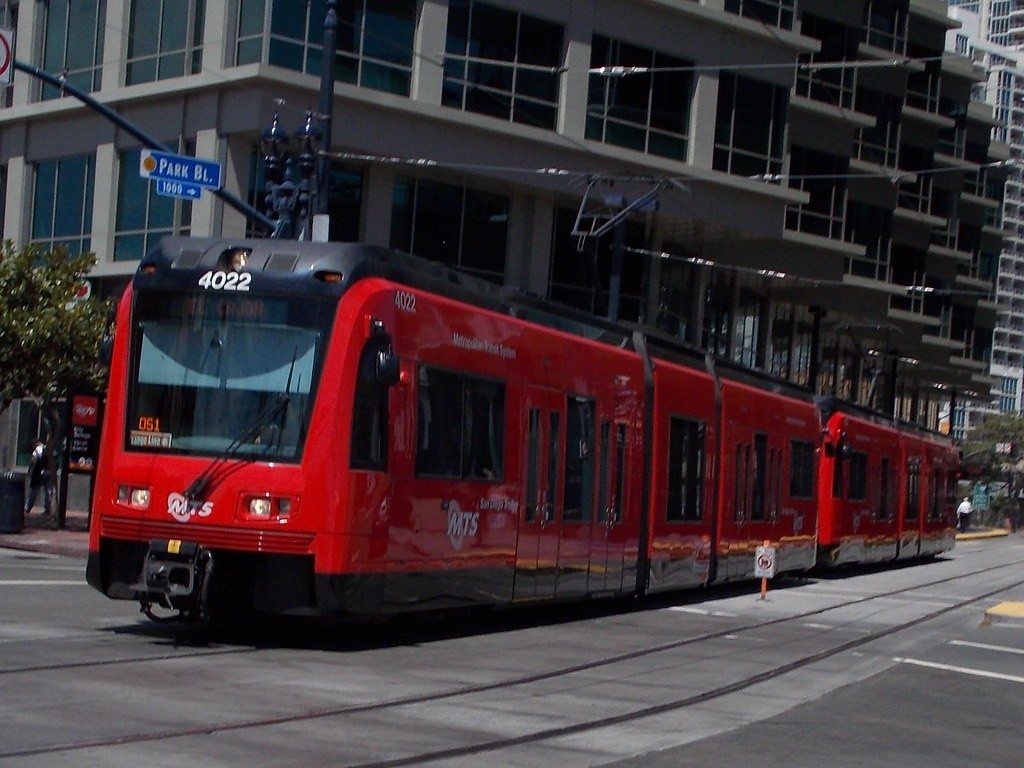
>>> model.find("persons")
[956,496,974,534]
[24,433,60,517]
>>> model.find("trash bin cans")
[0,473,27,534]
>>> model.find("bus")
[85,237,964,624]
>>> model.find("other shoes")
[41,511,50,516]
[25,504,30,514]
[961,529,965,533]
